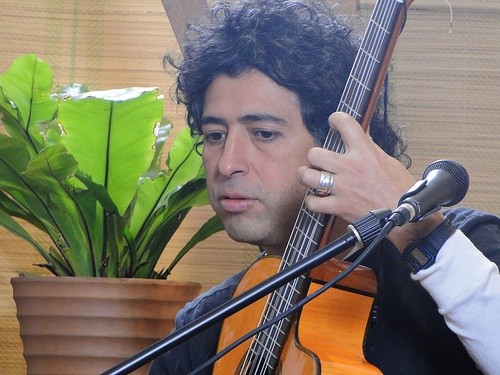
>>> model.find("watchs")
[401,216,458,275]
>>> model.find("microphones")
[391,159,470,227]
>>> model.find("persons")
[148,0,500,375]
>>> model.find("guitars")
[211,0,415,375]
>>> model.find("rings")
[316,171,334,197]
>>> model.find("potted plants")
[0,53,223,375]
